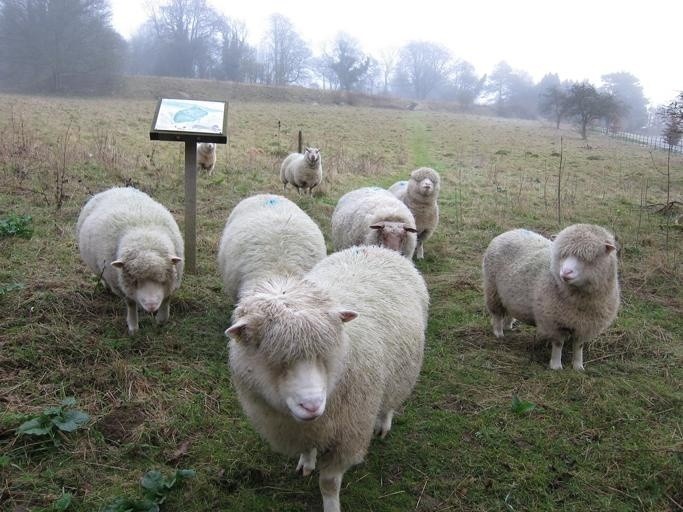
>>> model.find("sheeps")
[74,185,186,337]
[197,142,217,176]
[386,166,441,260]
[279,147,325,196]
[331,185,419,261]
[216,193,329,306]
[224,242,431,512]
[482,223,621,371]
